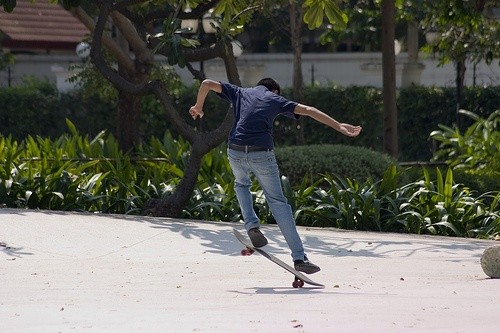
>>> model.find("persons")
[189,78,362,274]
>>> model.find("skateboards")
[232,228,325,288]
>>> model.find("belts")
[227,144,271,152]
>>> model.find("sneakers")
[248,228,268,247]
[294,260,320,274]
